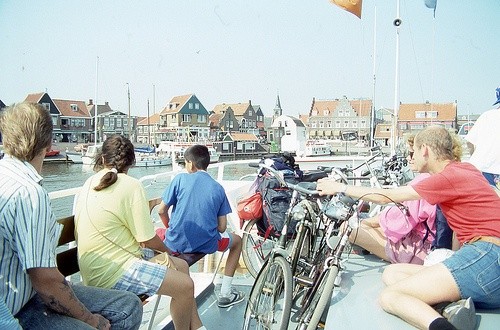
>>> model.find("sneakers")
[443,296,477,330]
[218,287,246,308]
[329,236,370,255]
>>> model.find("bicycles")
[240,141,413,330]
[242,161,331,330]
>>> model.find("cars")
[44,149,61,157]
[73,143,88,153]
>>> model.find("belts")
[469,235,500,247]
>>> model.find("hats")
[492,87,500,106]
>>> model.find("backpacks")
[255,170,301,233]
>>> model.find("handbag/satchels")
[148,251,178,271]
[237,192,263,219]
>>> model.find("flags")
[331,0,362,19]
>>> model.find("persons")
[73,134,207,330]
[156,146,245,308]
[0,102,144,330]
[315,88,500,330]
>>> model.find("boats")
[81,82,172,167]
[134,146,154,154]
[174,142,220,164]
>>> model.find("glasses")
[408,150,414,159]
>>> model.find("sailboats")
[65,55,101,163]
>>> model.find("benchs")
[56,198,225,330]
